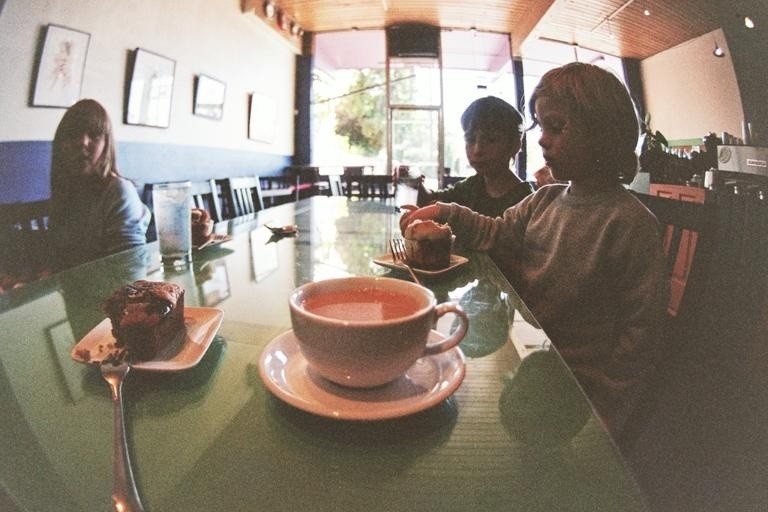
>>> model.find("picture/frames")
[193,73,227,122]
[124,46,177,129]
[31,22,91,109]
[248,91,276,144]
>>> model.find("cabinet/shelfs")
[649,182,716,320]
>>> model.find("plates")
[373,249,470,275]
[258,330,465,421]
[71,306,225,373]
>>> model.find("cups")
[153,182,193,273]
[288,276,470,389]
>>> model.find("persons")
[47,99,151,275]
[399,62,664,440]
[418,97,533,218]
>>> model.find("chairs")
[224,174,264,217]
[17,199,51,285]
[365,175,394,197]
[268,175,299,205]
[190,178,222,222]
[347,176,374,196]
[327,174,343,196]
[616,190,720,459]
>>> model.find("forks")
[198,234,223,250]
[389,238,420,284]
[99,349,145,511]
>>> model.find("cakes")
[192,209,215,247]
[102,279,188,363]
[404,218,458,269]
